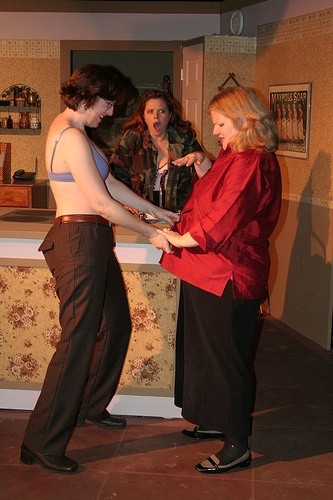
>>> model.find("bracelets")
[148,233,158,238]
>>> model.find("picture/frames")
[268,83,311,159]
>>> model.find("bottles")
[2,87,37,107]
[7,115,13,129]
[19,112,30,129]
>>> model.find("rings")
[166,228,170,233]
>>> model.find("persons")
[155,85,282,473]
[108,88,203,213]
[20,64,180,473]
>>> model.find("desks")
[0,207,185,419]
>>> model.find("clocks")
[230,10,243,35]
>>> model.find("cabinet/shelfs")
[0,177,49,208]
[0,85,41,135]
[80,83,160,156]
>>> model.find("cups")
[30,118,39,129]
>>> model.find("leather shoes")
[77,415,126,429]
[20,444,78,474]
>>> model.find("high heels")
[195,443,252,474]
[183,425,225,441]
